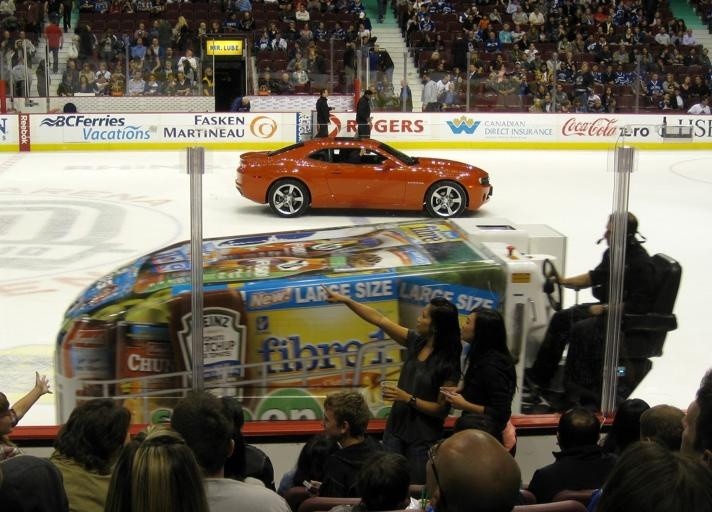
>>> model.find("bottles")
[229,235,377,262]
[206,253,381,273]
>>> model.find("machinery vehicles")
[53,219,684,427]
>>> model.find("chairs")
[0,0,712,117]
[280,464,601,512]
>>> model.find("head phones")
[626,232,647,246]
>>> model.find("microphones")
[596,237,605,244]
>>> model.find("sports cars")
[235,138,495,219]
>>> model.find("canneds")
[59,316,115,421]
[114,319,176,424]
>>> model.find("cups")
[382,382,396,405]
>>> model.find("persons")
[356,90,373,139]
[320,282,462,484]
[524,210,651,389]
[439,307,516,459]
[315,89,335,138]
[2,373,712,512]
[344,148,366,162]
[2,0,711,116]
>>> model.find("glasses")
[426,439,445,500]
